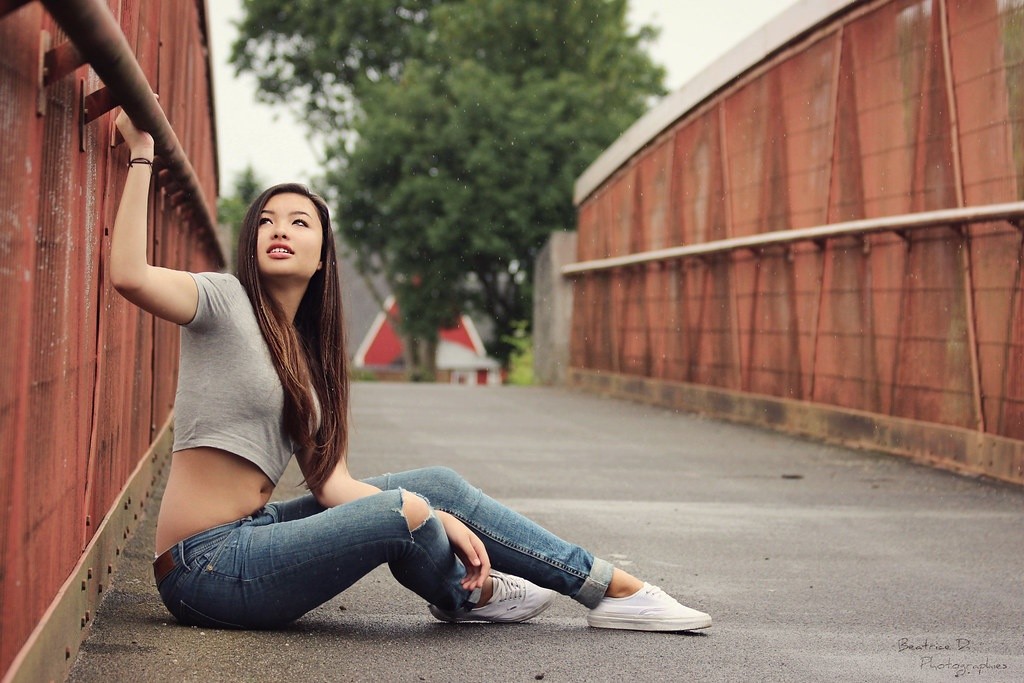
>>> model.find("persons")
[109,93,712,630]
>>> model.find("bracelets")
[126,157,153,172]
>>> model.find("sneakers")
[428,572,558,624]
[585,581,712,632]
